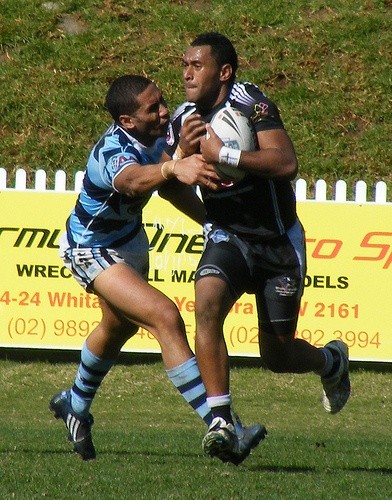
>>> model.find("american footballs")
[203,108,260,183]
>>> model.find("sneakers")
[48,391,98,462]
[230,423,268,467]
[320,340,351,415]
[201,416,237,463]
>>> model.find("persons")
[160,30,351,463]
[48,74,267,466]
